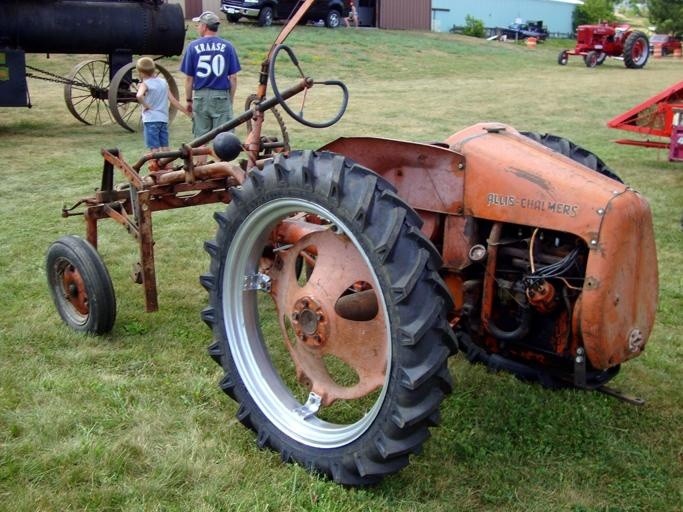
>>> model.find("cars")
[497,21,549,43]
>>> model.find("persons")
[178,10,241,165]
[343,2,358,29]
[135,57,193,164]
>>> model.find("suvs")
[220,0,352,28]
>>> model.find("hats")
[192,12,220,26]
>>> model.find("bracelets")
[185,99,192,102]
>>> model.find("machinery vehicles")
[45,0,660,489]
[558,20,650,68]
